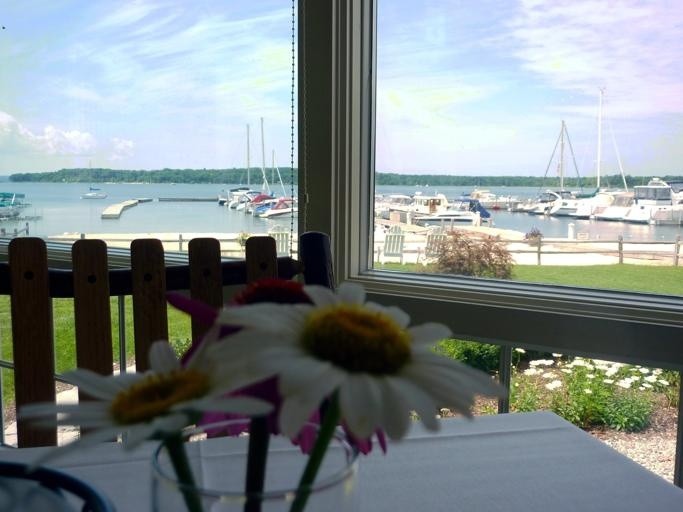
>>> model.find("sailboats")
[218,118,298,222]
[519,85,683,227]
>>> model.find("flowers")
[12,274,511,511]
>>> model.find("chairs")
[263,212,451,266]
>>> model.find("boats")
[82,191,107,200]
[0,192,31,219]
[373,187,521,234]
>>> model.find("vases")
[148,416,359,511]
